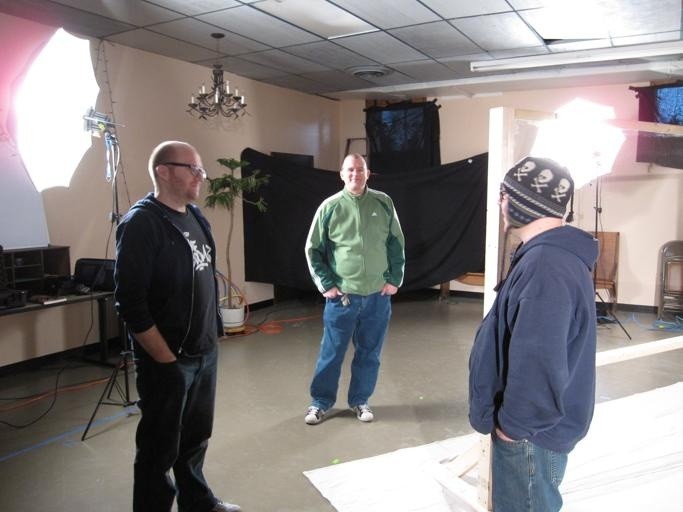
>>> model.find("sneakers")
[350,404,374,422]
[305,406,330,425]
[213,499,240,511]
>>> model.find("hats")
[501,156,574,229]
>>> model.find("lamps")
[186,31,251,122]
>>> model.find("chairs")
[587,231,619,321]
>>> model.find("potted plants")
[202,155,272,336]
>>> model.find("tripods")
[593,177,632,340]
[81,146,137,442]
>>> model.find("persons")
[111,139,245,511]
[302,151,407,425]
[466,155,598,511]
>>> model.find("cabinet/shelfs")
[0,244,122,368]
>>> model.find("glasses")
[162,162,207,179]
